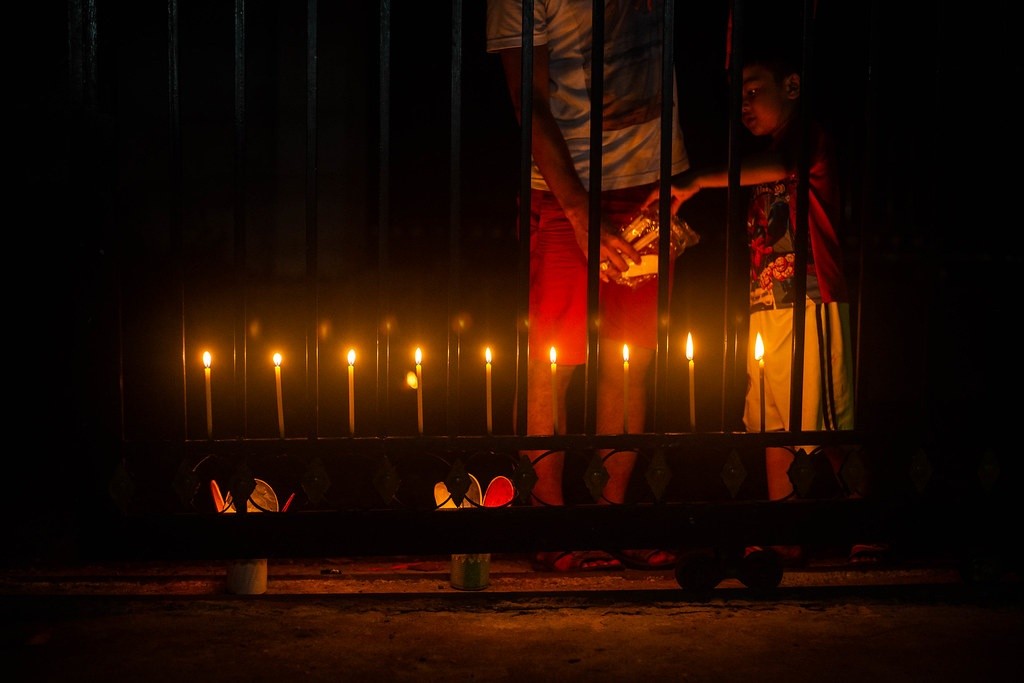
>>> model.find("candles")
[549,347,558,436]
[622,345,627,434]
[686,332,695,431]
[273,354,284,438]
[485,349,493,432]
[347,349,356,433]
[414,349,424,434]
[755,334,765,432]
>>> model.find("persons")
[641,45,890,571]
[486,0,690,572]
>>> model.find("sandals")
[619,549,677,569]
[532,550,625,573]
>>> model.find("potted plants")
[203,352,213,439]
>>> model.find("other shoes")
[848,544,883,568]
